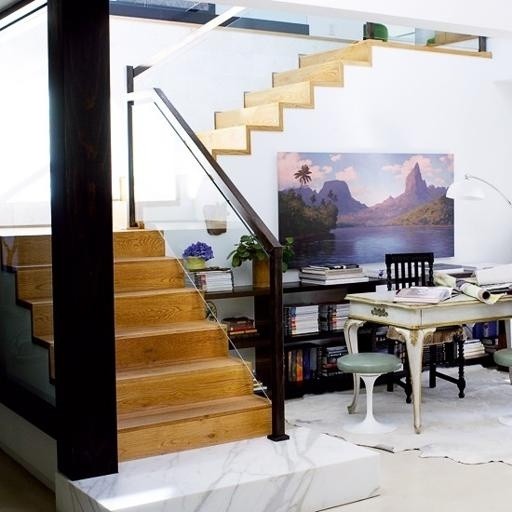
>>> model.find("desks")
[338,284,512,434]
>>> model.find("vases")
[187,257,206,270]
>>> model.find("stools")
[336,353,403,436]
[492,348,512,387]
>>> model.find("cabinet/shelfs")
[202,273,502,400]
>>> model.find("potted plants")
[230,231,295,287]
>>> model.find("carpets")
[283,363,512,467]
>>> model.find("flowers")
[179,242,215,261]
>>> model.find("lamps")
[446,163,512,210]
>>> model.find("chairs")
[384,252,468,404]
